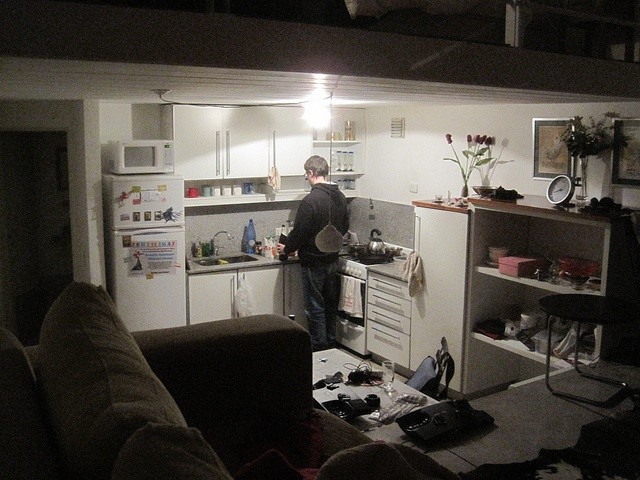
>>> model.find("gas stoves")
[336,255,406,280]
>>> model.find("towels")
[401,250,422,297]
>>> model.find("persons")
[277,156,349,350]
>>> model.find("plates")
[485,257,499,267]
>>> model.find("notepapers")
[146,273,155,279]
[133,199,140,205]
[158,184,166,191]
[132,186,141,193]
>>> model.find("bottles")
[303,177,312,192]
[279,224,288,261]
[575,157,589,200]
[337,180,343,189]
[349,180,356,190]
[344,120,353,141]
[246,219,256,255]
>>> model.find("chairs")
[538,217,637,407]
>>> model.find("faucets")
[211,231,234,255]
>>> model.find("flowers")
[443,131,493,184]
[562,112,615,160]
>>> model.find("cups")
[189,188,200,197]
[382,361,395,393]
[243,182,254,194]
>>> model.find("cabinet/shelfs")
[270,107,314,175]
[365,271,411,367]
[283,263,308,331]
[468,207,605,369]
[188,266,284,323]
[409,208,468,393]
[173,106,269,178]
[312,105,365,176]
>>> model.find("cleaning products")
[193,238,203,258]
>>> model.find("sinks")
[192,255,258,266]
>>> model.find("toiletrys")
[204,240,212,257]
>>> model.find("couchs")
[0,312,455,480]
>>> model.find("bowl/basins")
[488,245,511,254]
[488,252,510,263]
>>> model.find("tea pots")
[367,228,388,256]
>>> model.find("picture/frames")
[609,117,640,189]
[530,117,573,182]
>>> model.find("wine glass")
[336,150,342,171]
[348,152,354,171]
[343,151,348,171]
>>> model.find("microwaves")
[106,140,175,175]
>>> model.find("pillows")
[34,280,188,466]
[111,423,231,480]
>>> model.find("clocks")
[545,175,573,206]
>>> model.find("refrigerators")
[102,172,187,333]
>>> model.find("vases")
[460,186,469,198]
[582,162,588,200]
